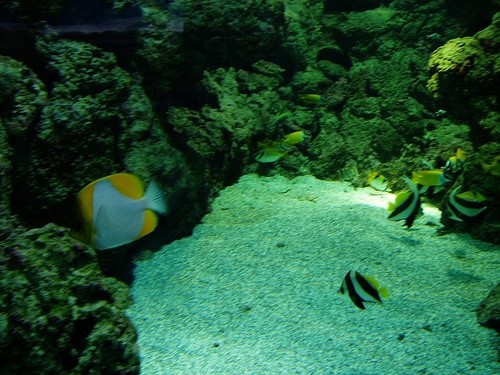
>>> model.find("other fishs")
[253,93,324,163]
[70,173,169,251]
[334,147,491,229]
[336,268,389,311]
